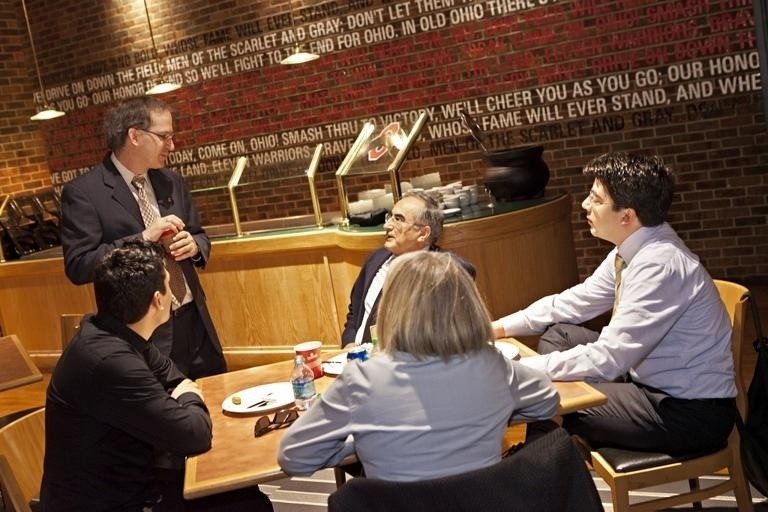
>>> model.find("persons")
[277,247,561,489]
[38,235,275,509]
[339,190,475,349]
[56,92,230,381]
[488,145,740,462]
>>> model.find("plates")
[222,381,296,414]
[324,348,346,376]
[490,341,519,360]
[438,208,462,217]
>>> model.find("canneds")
[160,225,179,261]
[346,348,367,363]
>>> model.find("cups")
[295,340,324,380]
[159,227,183,258]
[423,183,478,209]
[370,324,377,347]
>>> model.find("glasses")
[385,213,405,223]
[135,128,175,140]
[254,410,299,439]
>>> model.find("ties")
[612,254,628,318]
[129,176,187,307]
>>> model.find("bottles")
[290,355,316,409]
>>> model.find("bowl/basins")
[481,143,550,200]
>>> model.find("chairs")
[575,277,767,510]
[0,404,52,512]
[59,313,89,360]
[323,420,607,511]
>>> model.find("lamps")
[275,0,320,71]
[19,1,71,122]
[136,0,181,97]
